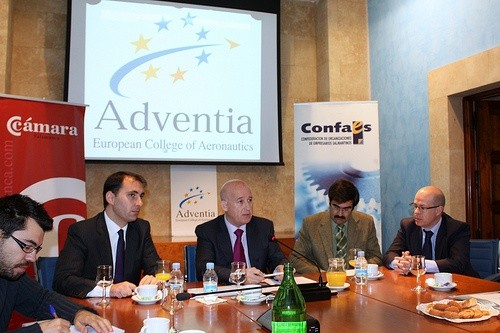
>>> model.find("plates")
[367,273,383,280]
[236,295,267,305]
[419,300,499,322]
[131,295,161,305]
[179,330,206,333]
[427,279,457,291]
[326,283,350,291]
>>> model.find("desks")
[20,267,500,333]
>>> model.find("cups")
[141,318,170,333]
[135,285,158,300]
[236,285,262,300]
[434,273,452,287]
[368,264,378,276]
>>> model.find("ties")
[113,229,126,284]
[232,229,247,270]
[335,224,347,269]
[421,229,434,261]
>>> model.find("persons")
[383,186,481,279]
[195,180,289,284]
[289,180,382,274]
[52,171,164,299]
[0,193,114,333]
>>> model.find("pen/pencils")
[264,272,284,277]
[49,304,59,318]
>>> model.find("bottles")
[356,251,367,285]
[272,264,307,333]
[203,263,218,302]
[169,263,184,290]
[327,258,346,287]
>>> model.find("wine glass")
[230,262,247,307]
[349,248,361,281]
[96,265,113,306]
[162,284,183,333]
[409,256,427,292]
[155,260,172,305]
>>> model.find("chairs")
[183,245,197,282]
[467,239,500,282]
[33,257,58,292]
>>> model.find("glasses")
[5,230,43,254]
[409,203,439,211]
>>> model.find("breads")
[427,297,489,319]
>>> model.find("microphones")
[268,233,331,302]
[176,284,280,301]
